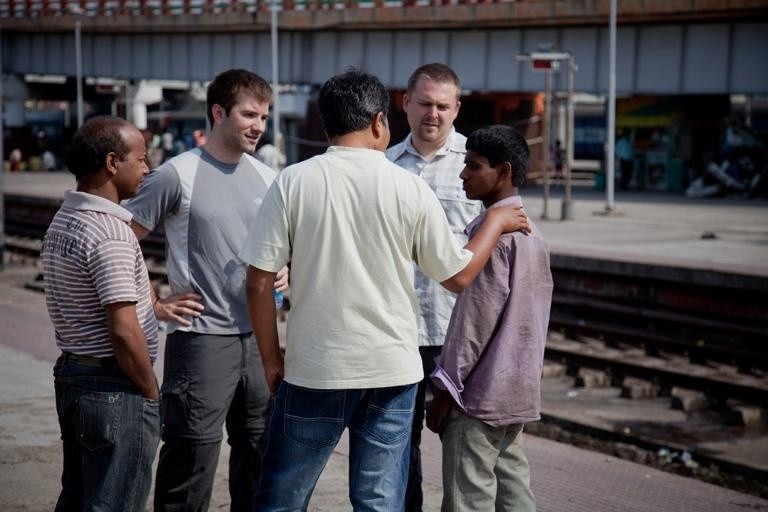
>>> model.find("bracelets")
[153,297,160,306]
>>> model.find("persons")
[122,70,290,512]
[553,140,564,178]
[40,115,164,512]
[237,68,532,512]
[425,124,555,512]
[10,127,286,176]
[383,62,486,512]
[615,126,637,191]
